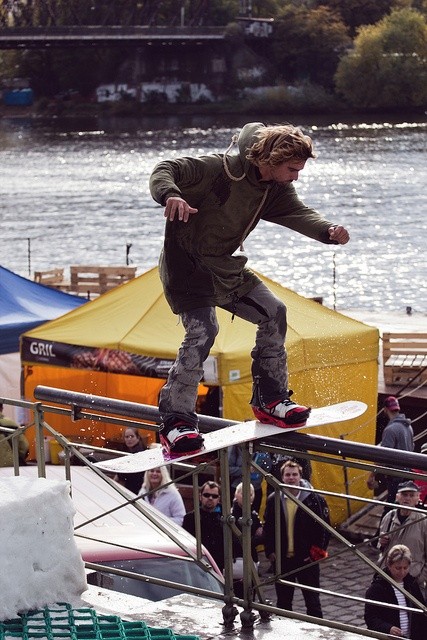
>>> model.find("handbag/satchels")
[223,558,257,580]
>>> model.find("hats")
[385,395,400,411]
[397,480,420,493]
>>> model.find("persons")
[112,427,150,495]
[363,544,426,640]
[136,465,186,527]
[262,431,312,524]
[400,443,426,504]
[0,403,28,468]
[261,460,330,619]
[362,396,414,552]
[376,480,426,603]
[148,122,350,451]
[181,481,224,576]
[226,419,262,516]
[230,482,260,607]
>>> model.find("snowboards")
[89,400,369,473]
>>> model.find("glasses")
[202,493,220,498]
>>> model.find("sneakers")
[159,424,203,454]
[251,399,308,424]
